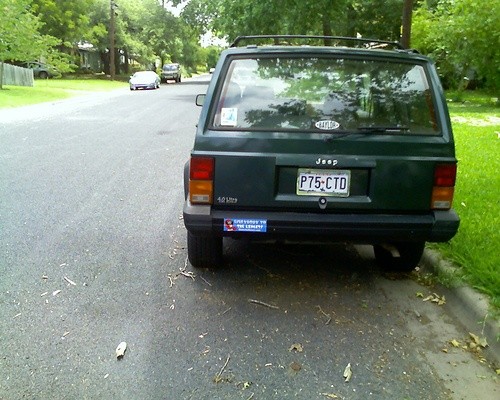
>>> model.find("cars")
[17,61,58,79]
[129,71,160,89]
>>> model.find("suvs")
[182,34,460,273]
[161,64,181,83]
